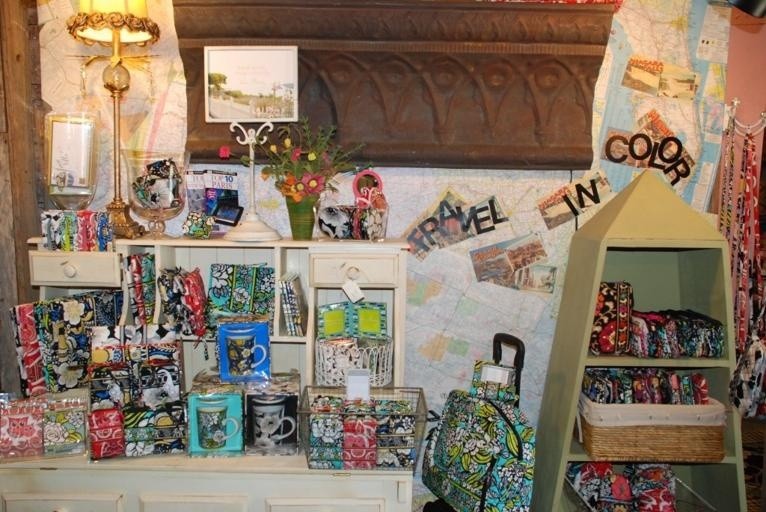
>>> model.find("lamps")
[66,1,160,241]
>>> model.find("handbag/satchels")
[421,358,537,511]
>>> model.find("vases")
[284,194,319,240]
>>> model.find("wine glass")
[119,149,191,240]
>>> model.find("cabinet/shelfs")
[530,169,748,512]
[0,236,413,512]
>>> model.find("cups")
[8,416,28,438]
[253,405,296,447]
[225,336,267,375]
[196,406,239,449]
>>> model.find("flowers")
[220,116,375,202]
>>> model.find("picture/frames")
[204,45,299,123]
[39,110,98,196]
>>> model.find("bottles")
[42,110,98,211]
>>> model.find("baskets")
[297,386,427,470]
[313,332,394,389]
